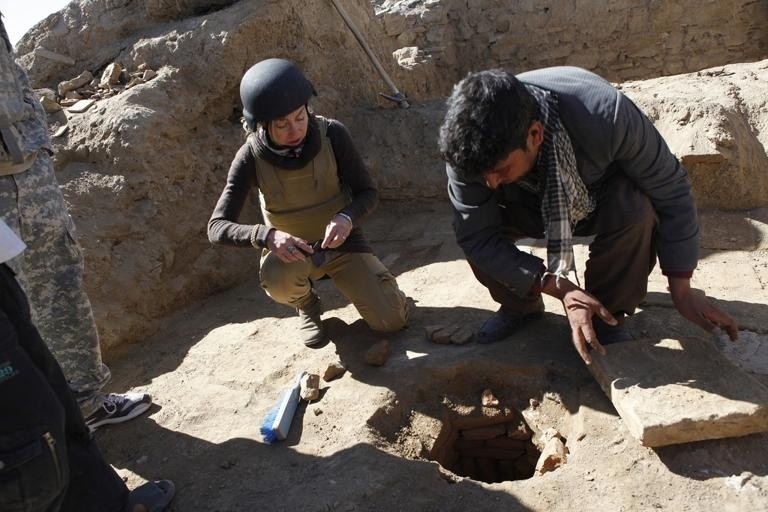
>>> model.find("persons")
[206,58,408,348]
[437,66,738,367]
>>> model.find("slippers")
[129,479,176,511]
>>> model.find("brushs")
[260,371,305,444]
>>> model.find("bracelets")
[336,213,353,229]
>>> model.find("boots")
[292,288,325,345]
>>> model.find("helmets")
[240,57,318,132]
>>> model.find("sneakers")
[73,390,152,434]
[597,315,634,344]
[474,295,544,345]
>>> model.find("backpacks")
[1,409,73,512]
